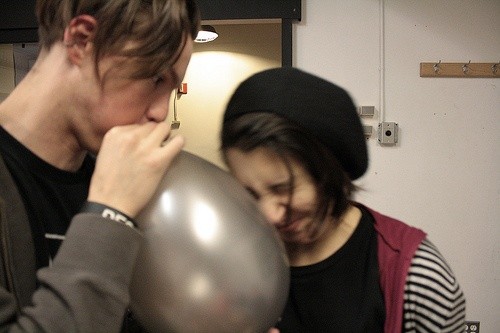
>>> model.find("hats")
[223,65,369,181]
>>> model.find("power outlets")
[465,321,478,333]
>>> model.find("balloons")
[128,148,290,333]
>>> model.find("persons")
[219,65,468,333]
[0,0,282,333]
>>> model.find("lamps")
[192,25,219,44]
